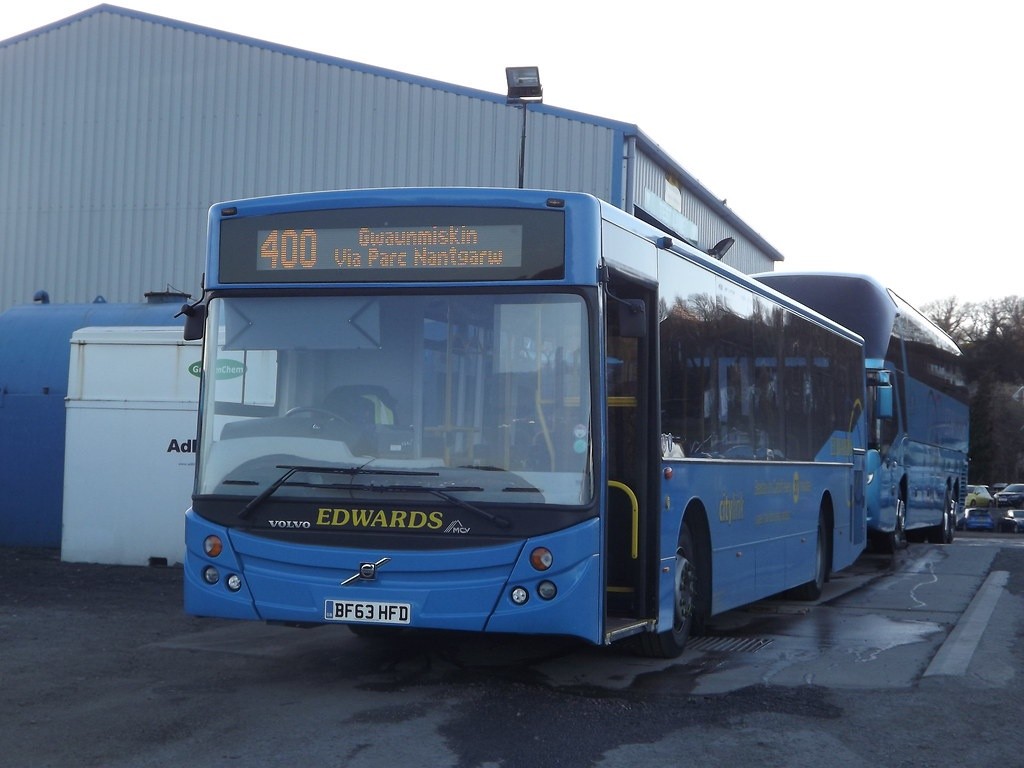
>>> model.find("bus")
[745,269,972,556]
[173,185,870,660]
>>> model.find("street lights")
[504,65,544,188]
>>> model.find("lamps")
[505,66,542,98]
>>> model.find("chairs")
[326,385,389,426]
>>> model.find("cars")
[993,482,1007,490]
[964,486,994,508]
[955,507,994,533]
[993,484,1024,508]
[998,509,1024,532]
